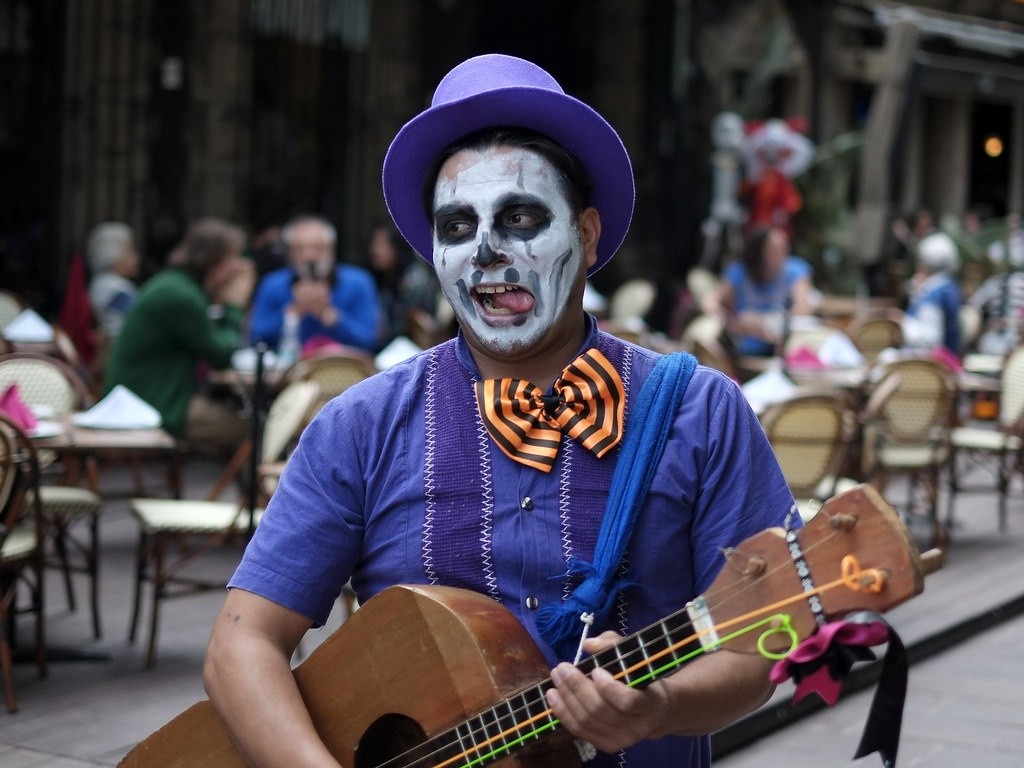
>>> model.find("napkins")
[822,328,862,369]
[1,306,57,340]
[70,385,163,429]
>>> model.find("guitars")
[117,483,925,768]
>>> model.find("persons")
[202,53,804,768]
[80,201,1024,489]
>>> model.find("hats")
[380,55,634,279]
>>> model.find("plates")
[20,422,66,438]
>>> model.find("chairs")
[0,292,450,713]
[605,291,1024,549]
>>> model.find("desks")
[203,369,278,498]
[5,409,176,665]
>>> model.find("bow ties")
[474,349,624,475]
